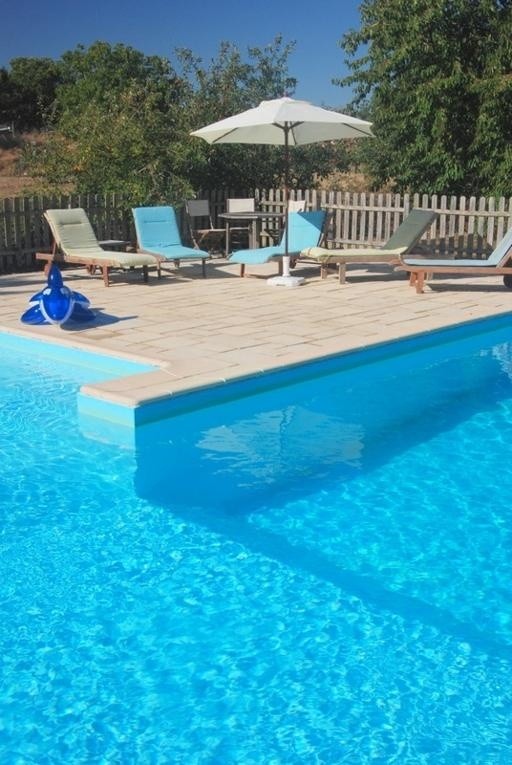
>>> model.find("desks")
[218,210,285,255]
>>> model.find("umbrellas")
[187,92,380,260]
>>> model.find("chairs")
[227,198,256,214]
[304,209,437,285]
[187,199,226,254]
[131,206,211,282]
[231,209,330,280]
[260,199,307,246]
[42,208,157,289]
[394,227,512,293]
